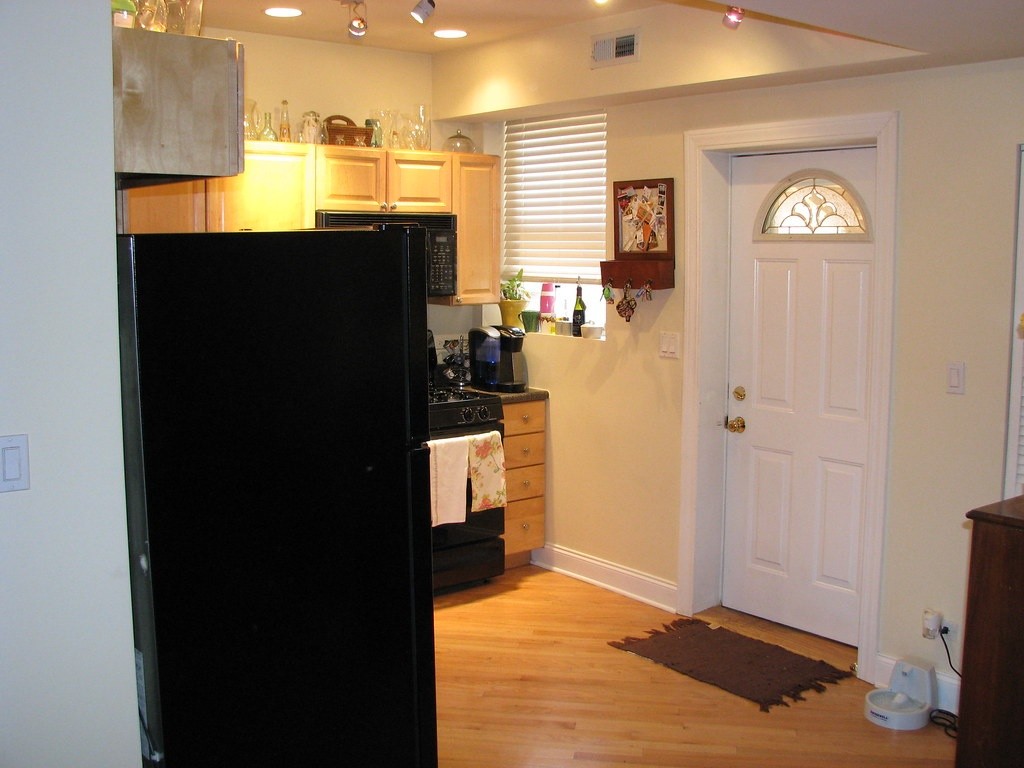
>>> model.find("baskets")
[323,114,374,147]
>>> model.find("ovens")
[431,425,506,596]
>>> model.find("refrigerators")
[115,221,440,768]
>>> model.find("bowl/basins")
[443,129,476,154]
[580,323,603,339]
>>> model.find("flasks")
[539,284,554,313]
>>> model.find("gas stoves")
[429,331,504,430]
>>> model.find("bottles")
[258,112,277,142]
[571,285,586,337]
[243,97,259,141]
[279,99,291,142]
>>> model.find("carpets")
[605,618,853,716]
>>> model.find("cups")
[300,111,323,143]
[517,310,540,332]
[111,0,204,37]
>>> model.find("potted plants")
[497,267,530,330]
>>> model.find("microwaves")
[315,209,458,297]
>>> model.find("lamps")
[340,0,368,40]
[410,0,436,23]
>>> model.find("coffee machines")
[468,325,529,393]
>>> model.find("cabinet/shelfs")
[112,25,246,177]
[953,494,1024,767]
[116,138,505,307]
[499,399,549,572]
